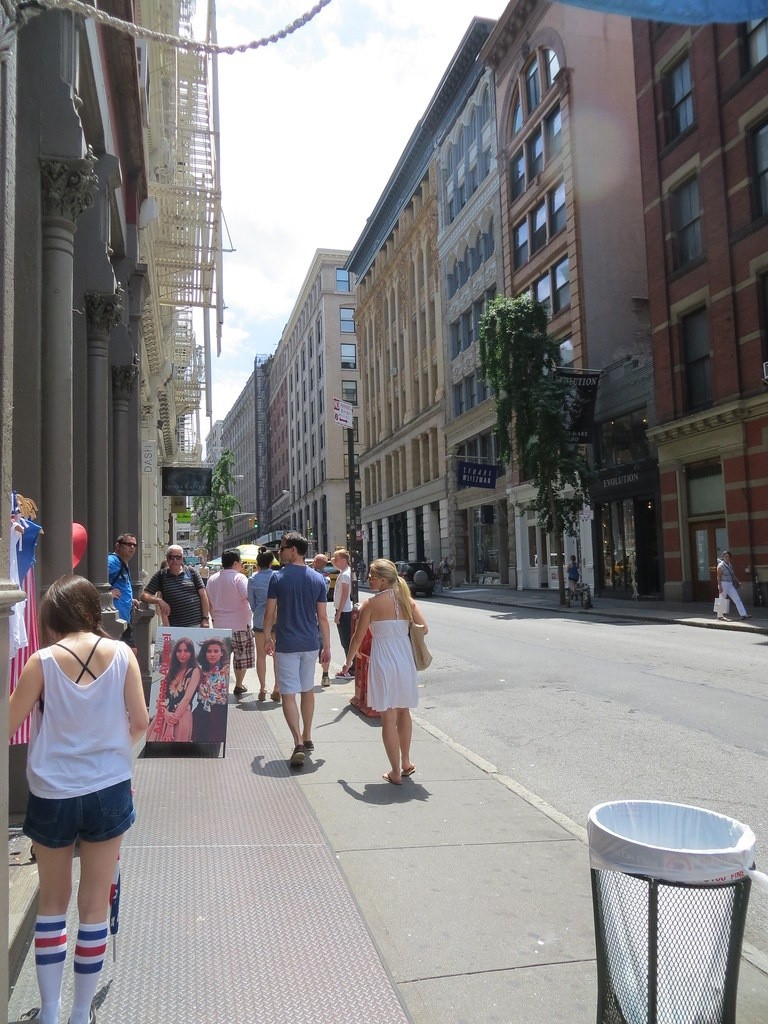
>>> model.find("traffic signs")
[332,397,353,429]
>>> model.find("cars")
[304,558,342,600]
[610,556,633,587]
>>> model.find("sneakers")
[336,670,356,679]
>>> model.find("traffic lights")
[254,518,258,529]
[249,518,252,528]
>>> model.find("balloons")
[72,522,87,569]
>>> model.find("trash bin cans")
[586,800,757,1024]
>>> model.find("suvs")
[394,560,436,598]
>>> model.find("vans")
[534,553,564,568]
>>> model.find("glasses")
[369,574,380,581]
[169,555,182,560]
[280,545,292,552]
[120,542,138,547]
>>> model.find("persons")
[717,551,751,621]
[146,638,230,741]
[424,557,453,592]
[342,558,428,785]
[263,533,330,768]
[567,555,579,601]
[8,574,149,1024]
[107,532,355,702]
[356,559,368,585]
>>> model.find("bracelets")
[345,660,353,667]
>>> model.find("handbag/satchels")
[733,580,739,588]
[713,591,730,614]
[409,618,433,671]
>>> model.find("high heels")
[271,691,281,701]
[259,691,266,701]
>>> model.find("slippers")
[383,772,402,784]
[401,764,416,775]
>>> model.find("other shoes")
[718,616,726,620]
[16,1007,41,1024]
[303,741,314,750]
[742,614,751,619]
[234,685,247,694]
[290,745,306,766]
[321,676,330,686]
[67,1007,96,1023]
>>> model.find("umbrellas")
[109,789,136,961]
